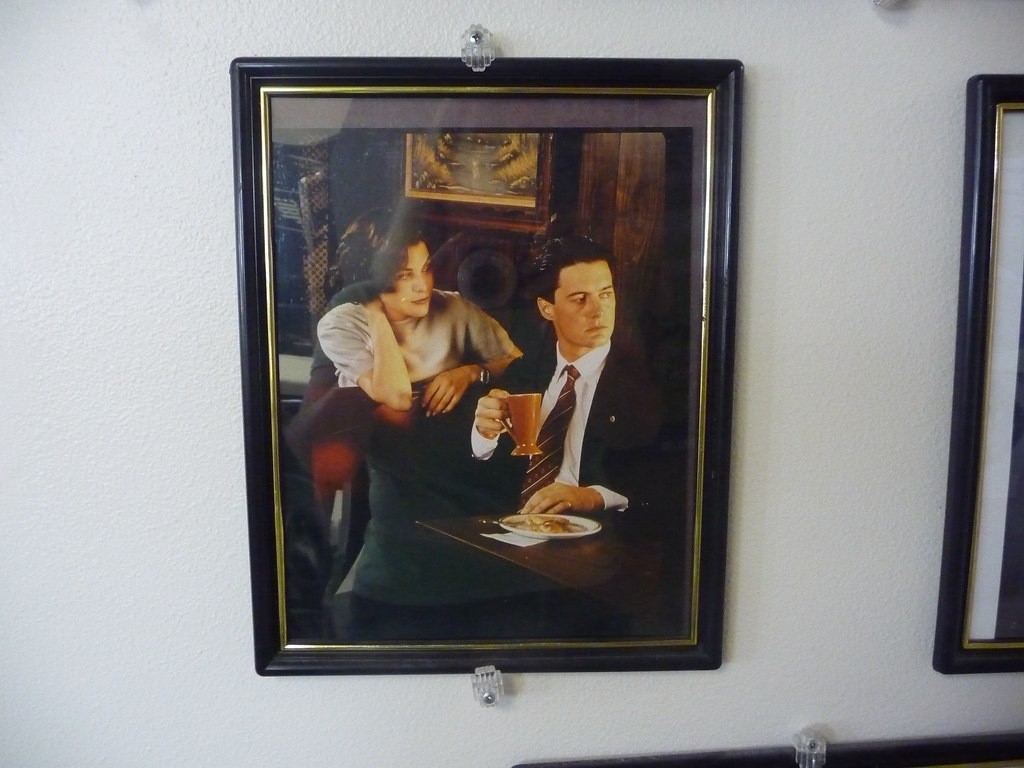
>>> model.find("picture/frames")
[227,55,745,677]
[390,131,560,231]
[931,73,1024,676]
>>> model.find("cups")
[499,394,543,456]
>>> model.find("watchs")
[479,365,490,384]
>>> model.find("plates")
[499,514,601,538]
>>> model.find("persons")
[316,207,525,418]
[467,236,676,515]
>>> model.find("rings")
[568,502,571,509]
